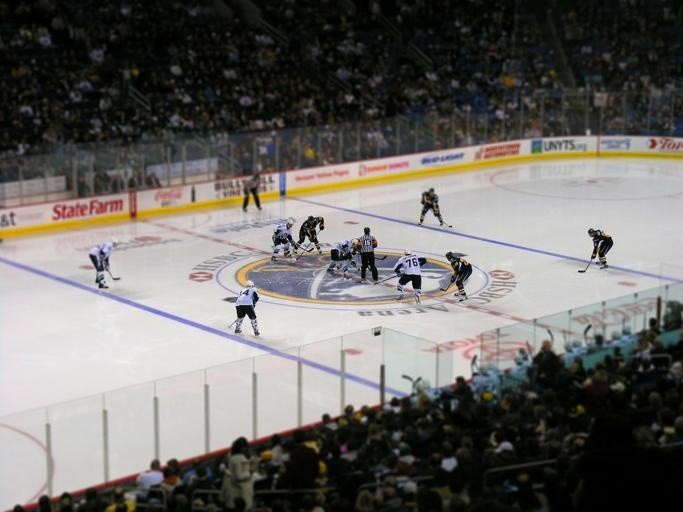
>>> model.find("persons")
[292,215,324,258]
[444,251,472,302]
[326,237,360,279]
[0,301,681,510]
[88,237,119,273]
[417,188,444,228]
[585,228,614,270]
[393,247,426,306]
[0,1,683,198]
[357,226,379,283]
[241,172,263,212]
[270,218,300,263]
[231,278,263,338]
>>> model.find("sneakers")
[596,262,608,269]
[455,293,468,302]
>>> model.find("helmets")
[404,249,411,255]
[364,227,370,235]
[288,219,293,224]
[245,280,254,287]
[588,229,595,237]
[446,252,454,259]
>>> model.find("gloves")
[451,276,456,282]
[591,253,596,259]
[320,223,324,230]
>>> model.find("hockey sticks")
[296,230,321,260]
[375,255,388,261]
[440,282,451,292]
[578,259,591,273]
[470,355,477,375]
[373,274,397,285]
[108,269,120,280]
[584,323,592,347]
[439,216,453,227]
[402,374,423,396]
[547,330,554,346]
[299,246,314,253]
